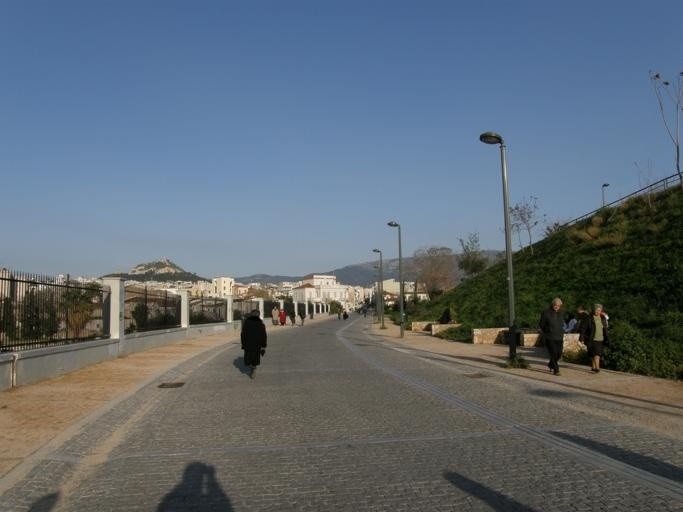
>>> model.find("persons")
[271,306,279,325]
[277,308,287,326]
[580,303,610,372]
[571,300,590,333]
[299,306,305,326]
[342,309,348,320]
[240,309,266,378]
[287,307,295,325]
[336,308,340,319]
[561,307,577,335]
[363,306,367,317]
[540,298,564,376]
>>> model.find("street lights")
[372,265,381,323]
[371,248,387,330]
[476,129,516,330]
[386,219,406,338]
[601,183,610,208]
